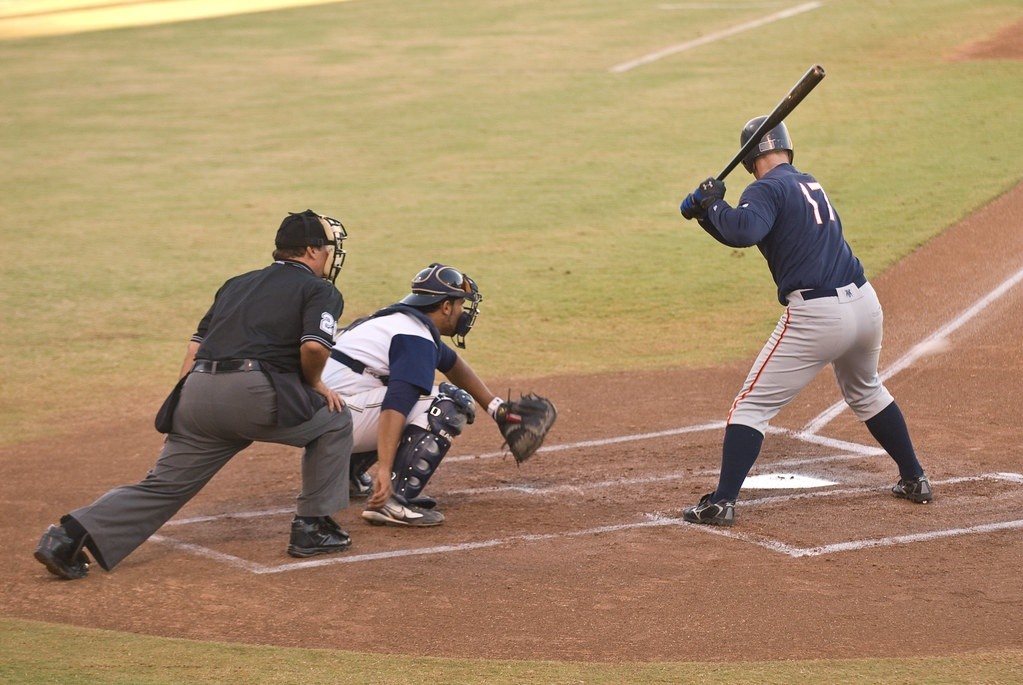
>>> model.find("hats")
[275,211,336,246]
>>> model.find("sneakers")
[891,479,933,504]
[34,523,88,580]
[362,497,445,527]
[288,519,352,558]
[682,491,734,526]
[349,475,374,497]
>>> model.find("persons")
[33,212,363,582]
[681,115,936,525]
[313,263,545,528]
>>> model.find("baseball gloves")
[495,399,557,463]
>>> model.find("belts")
[193,360,262,373]
[800,274,867,300]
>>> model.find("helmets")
[739,116,794,173]
[400,263,466,307]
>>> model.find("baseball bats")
[681,65,826,220]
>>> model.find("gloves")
[694,177,726,209]
[679,192,707,220]
